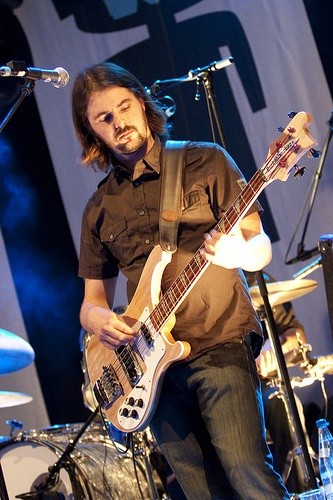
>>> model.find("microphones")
[146,80,162,96]
[6,420,23,430]
[286,247,320,265]
[19,491,65,500]
[0,66,70,89]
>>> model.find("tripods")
[255,305,320,494]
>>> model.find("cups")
[298,489,325,500]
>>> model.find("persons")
[243,269,306,493]
[71,62,289,500]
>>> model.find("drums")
[78,304,131,421]
[24,422,109,439]
[1,436,166,500]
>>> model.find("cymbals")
[248,279,320,311]
[0,391,33,408]
[0,328,36,376]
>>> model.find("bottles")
[315,419,333,500]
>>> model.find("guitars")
[81,109,319,434]
